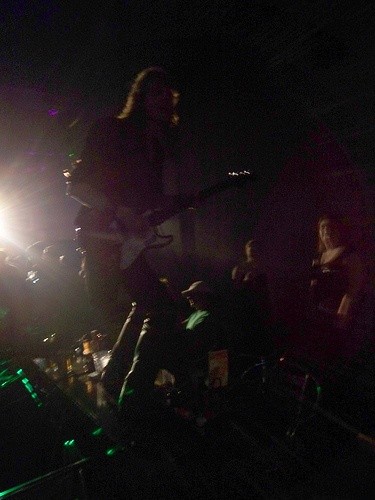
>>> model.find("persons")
[72,67,182,398]
[306,213,362,361]
[231,239,274,336]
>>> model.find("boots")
[102,300,141,404]
[103,321,162,424]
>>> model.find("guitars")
[73,169,257,275]
